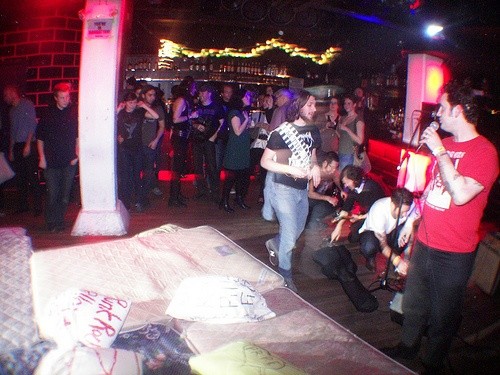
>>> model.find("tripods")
[369,122,420,295]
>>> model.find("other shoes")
[210,185,218,196]
[258,198,264,202]
[379,341,419,359]
[193,186,205,198]
[283,276,297,292]
[265,239,279,266]
[151,187,164,195]
[220,199,232,211]
[237,198,247,209]
[135,203,144,212]
[48,222,65,233]
[168,199,188,208]
[179,194,187,202]
[366,258,376,272]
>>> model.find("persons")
[306,151,347,230]
[317,88,369,170]
[358,187,416,274]
[260,88,321,291]
[3,80,44,217]
[35,82,79,232]
[397,81,500,375]
[117,76,296,215]
[330,166,384,274]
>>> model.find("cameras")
[329,122,336,127]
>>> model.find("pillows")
[34,346,144,375]
[188,340,309,375]
[61,289,132,348]
[166,275,276,323]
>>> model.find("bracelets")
[393,256,400,266]
[432,146,448,157]
[187,116,189,120]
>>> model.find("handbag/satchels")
[353,151,371,175]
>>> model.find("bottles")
[124,54,290,86]
[305,64,406,129]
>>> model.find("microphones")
[415,122,439,153]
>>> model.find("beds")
[0,225,416,375]
